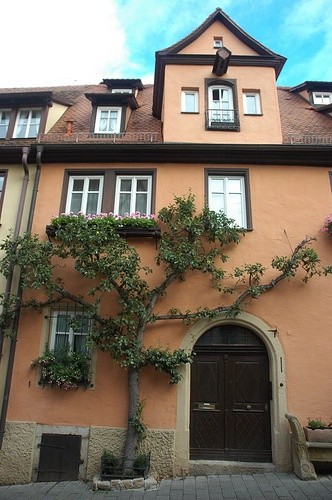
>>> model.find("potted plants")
[303,419,332,443]
